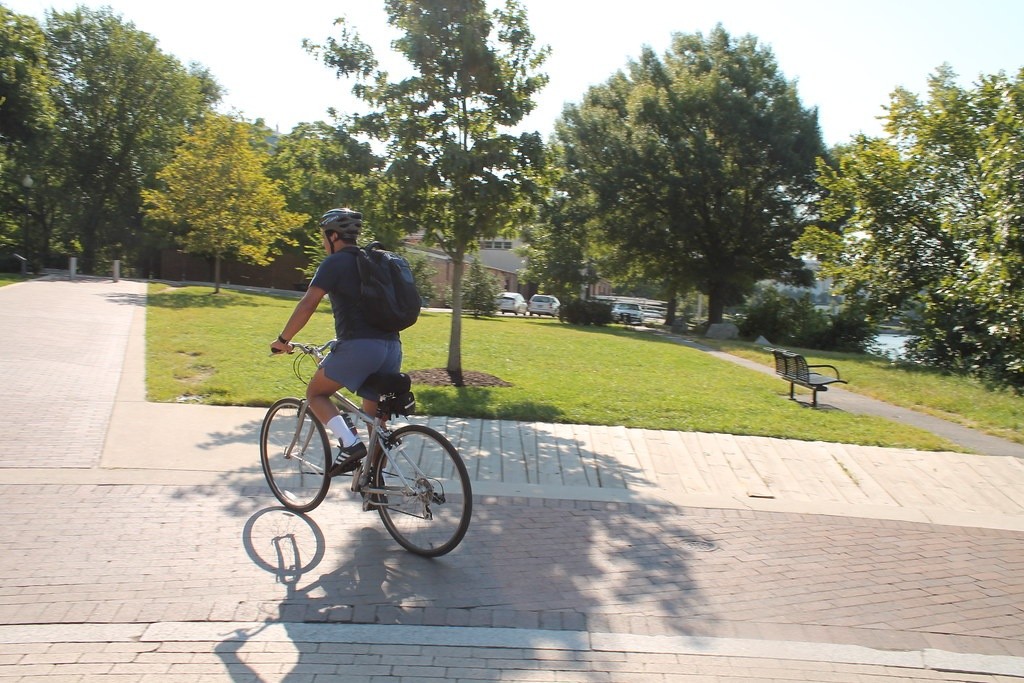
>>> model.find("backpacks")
[337,241,422,331]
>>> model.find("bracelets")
[278,334,290,346]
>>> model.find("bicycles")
[260,339,472,557]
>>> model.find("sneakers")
[328,438,368,477]
[367,494,388,511]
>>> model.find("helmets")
[319,208,363,238]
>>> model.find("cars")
[527,293,560,318]
[610,303,644,325]
[496,292,528,315]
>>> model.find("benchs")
[764,346,847,407]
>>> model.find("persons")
[271,208,404,510]
